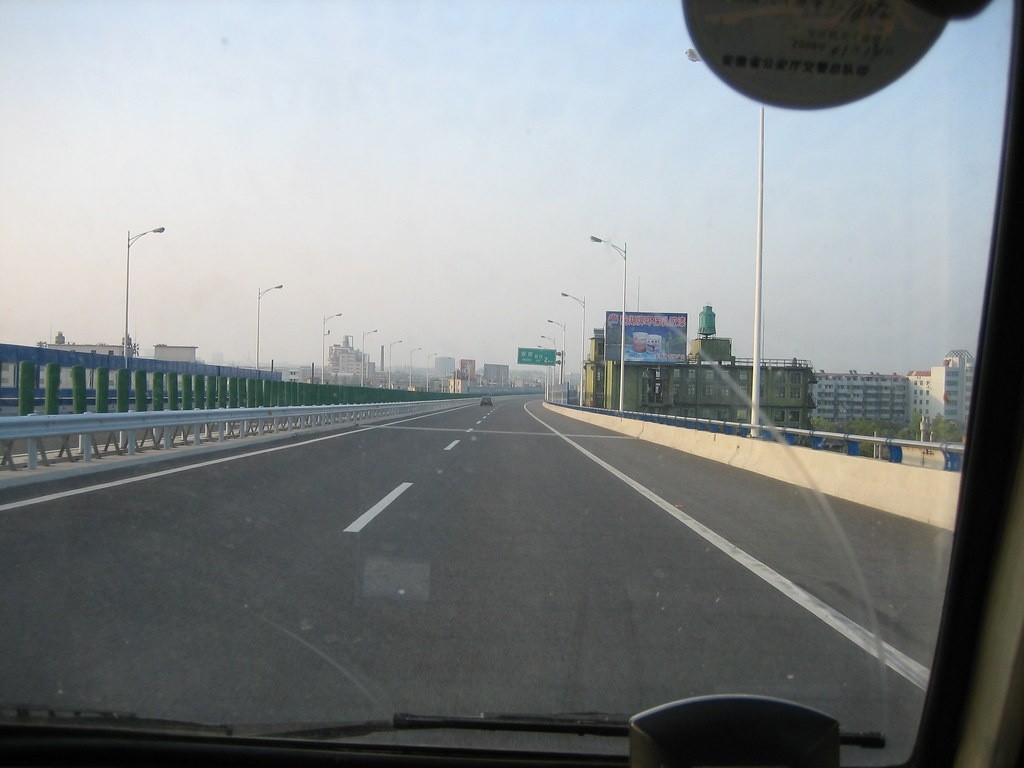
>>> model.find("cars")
[479,396,494,406]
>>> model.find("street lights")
[410,347,421,388]
[561,292,586,406]
[321,314,343,384]
[122,226,166,366]
[541,335,556,402]
[257,285,283,369]
[361,330,378,388]
[590,235,628,409]
[389,340,403,389]
[548,320,566,403]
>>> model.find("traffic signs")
[516,346,557,366]
